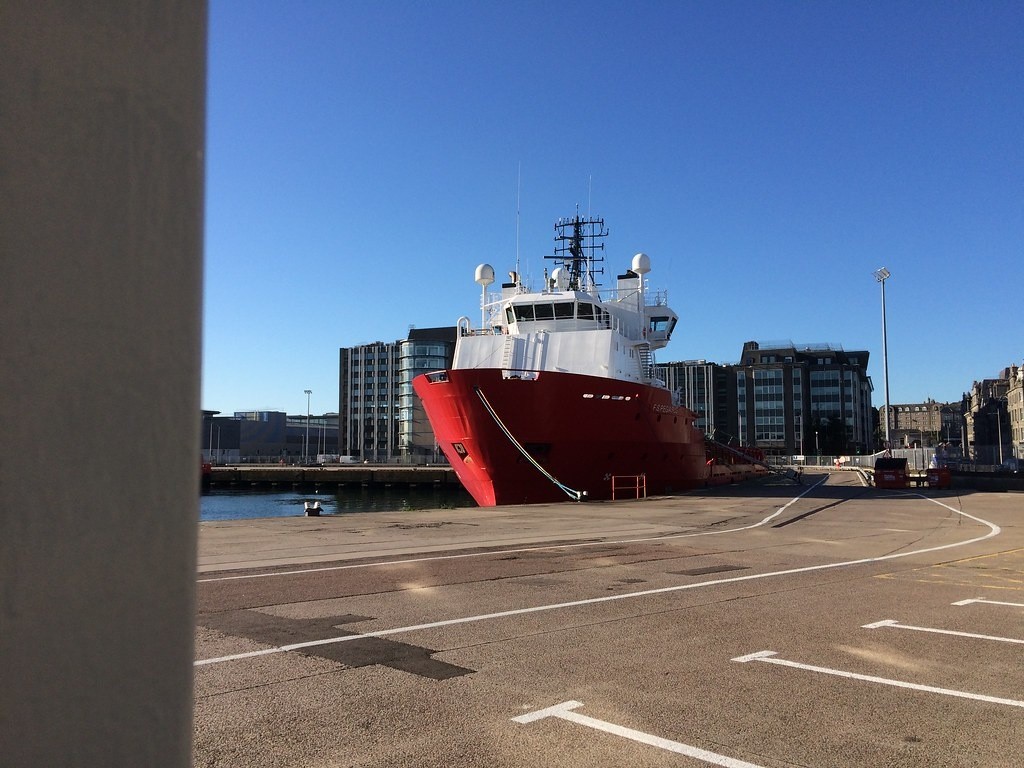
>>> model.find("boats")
[408,202,778,508]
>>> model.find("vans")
[340,456,357,464]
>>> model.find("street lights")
[815,432,819,451]
[986,408,1002,466]
[210,422,215,461]
[216,424,221,465]
[304,389,312,462]
[871,267,892,455]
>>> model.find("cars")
[995,458,1024,478]
